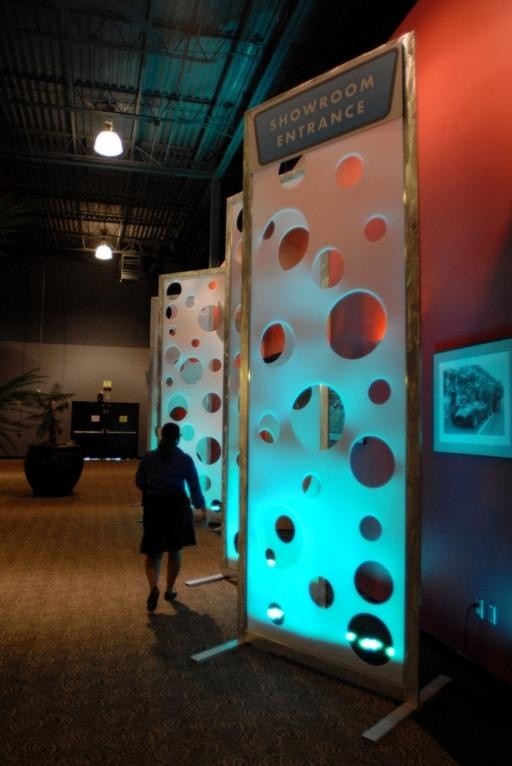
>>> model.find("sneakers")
[148,587,159,611]
[165,591,176,600]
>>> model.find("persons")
[136,423,208,611]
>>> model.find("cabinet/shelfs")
[71,400,140,460]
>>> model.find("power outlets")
[471,596,487,620]
[486,604,498,627]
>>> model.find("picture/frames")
[429,333,511,461]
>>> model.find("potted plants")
[19,379,85,498]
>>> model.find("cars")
[454,400,490,429]
